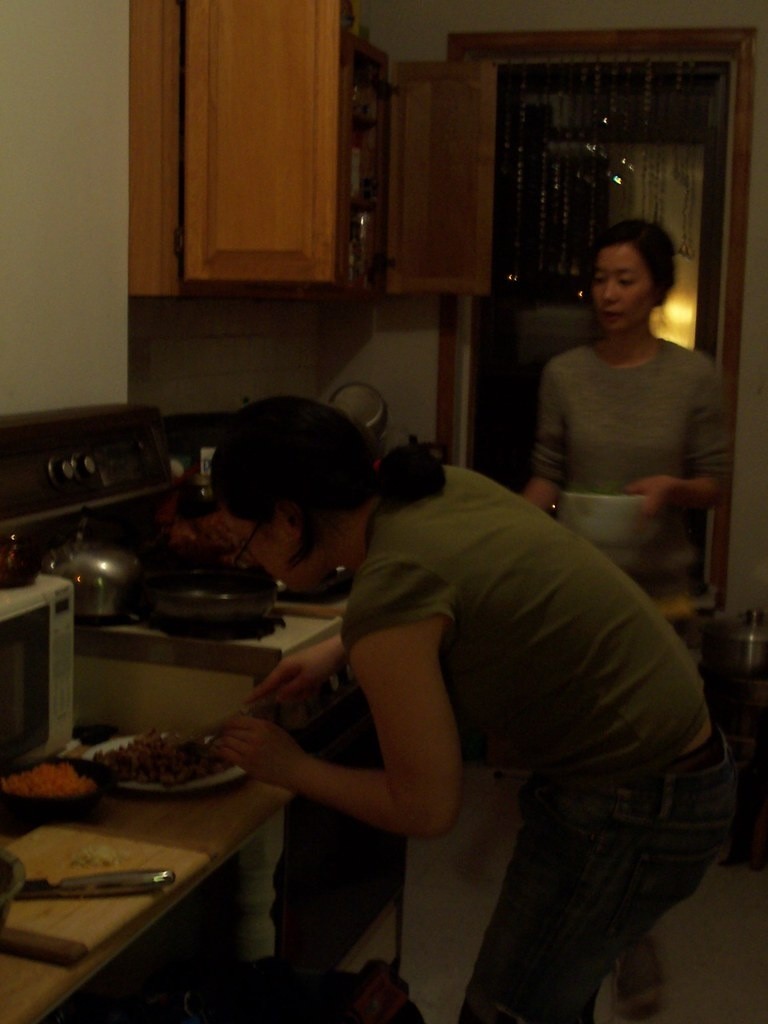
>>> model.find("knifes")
[16,869,176,900]
[198,692,287,738]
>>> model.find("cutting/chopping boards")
[0,825,213,964]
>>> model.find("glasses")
[234,515,269,569]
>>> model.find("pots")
[40,506,279,633]
[700,606,768,675]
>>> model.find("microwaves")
[0,574,73,763]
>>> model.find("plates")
[82,735,251,793]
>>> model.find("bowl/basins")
[556,491,652,551]
[0,847,25,936]
[328,383,388,444]
[0,757,117,827]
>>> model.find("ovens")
[231,627,413,981]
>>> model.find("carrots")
[0,761,98,797]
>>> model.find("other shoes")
[610,921,664,1019]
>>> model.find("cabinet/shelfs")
[129,0,494,302]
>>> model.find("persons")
[210,390,736,1024]
[520,217,730,678]
